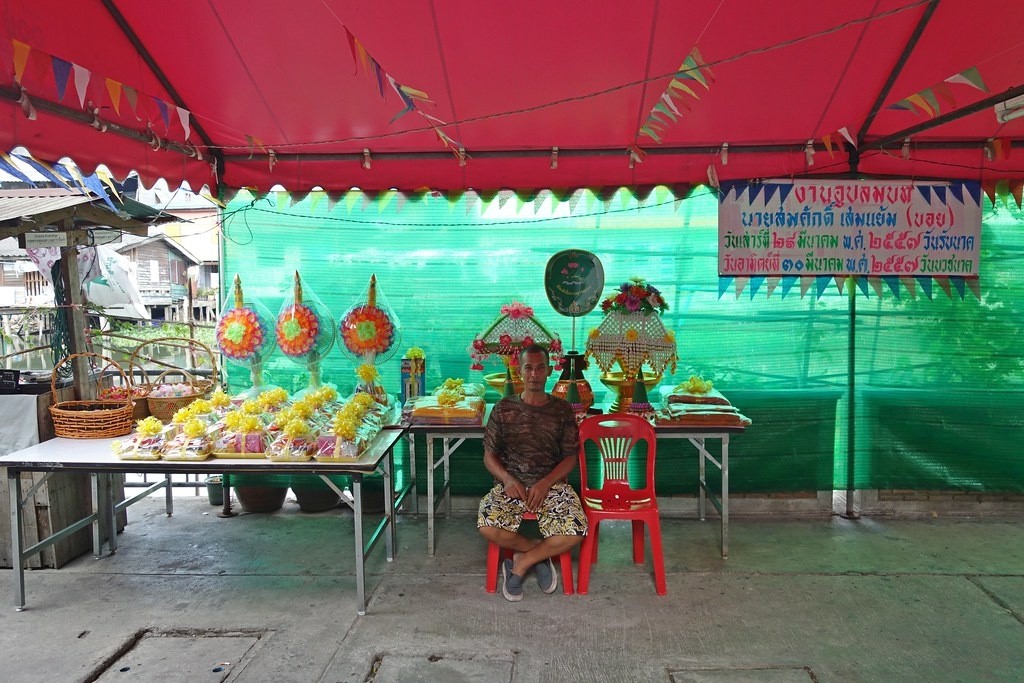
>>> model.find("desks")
[0,402,745,616]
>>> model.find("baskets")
[97,358,153,420]
[144,368,206,424]
[128,337,217,404]
[47,351,134,438]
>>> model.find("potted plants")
[205,476,223,506]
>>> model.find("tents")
[0,0,1024,520]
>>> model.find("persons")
[476,343,588,602]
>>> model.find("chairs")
[486,511,575,595]
[577,414,667,596]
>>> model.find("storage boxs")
[401,355,426,407]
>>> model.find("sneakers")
[502,559,524,601]
[533,556,558,594]
